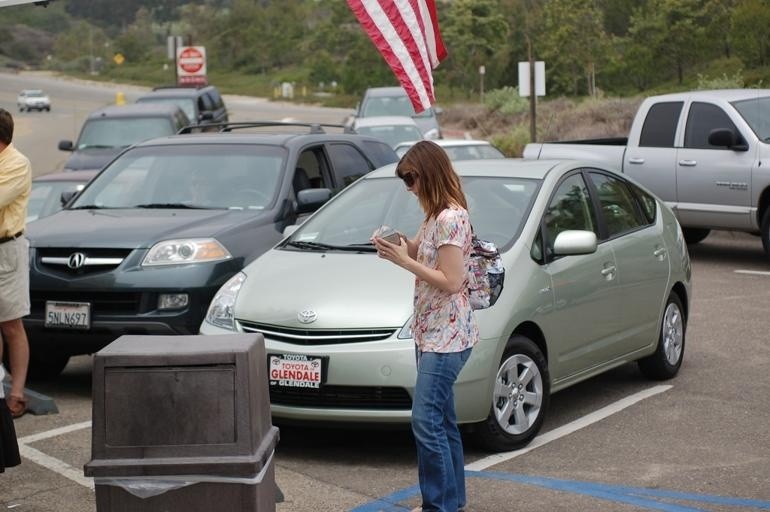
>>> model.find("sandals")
[6,395,26,418]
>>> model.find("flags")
[345,0,448,115]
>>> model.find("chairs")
[293,168,313,214]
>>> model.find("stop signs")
[177,48,206,75]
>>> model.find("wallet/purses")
[375,225,408,256]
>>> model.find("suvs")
[3,120,401,384]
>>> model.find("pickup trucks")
[522,87,769,259]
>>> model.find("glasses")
[403,172,420,188]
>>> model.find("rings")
[382,251,385,256]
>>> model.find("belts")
[0,232,23,245]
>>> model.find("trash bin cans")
[83,333,280,512]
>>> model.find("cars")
[200,158,693,453]
[18,89,51,113]
[347,83,504,164]
[25,82,236,219]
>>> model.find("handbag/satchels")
[468,224,506,311]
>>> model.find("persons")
[1,107,32,418]
[371,140,478,512]
